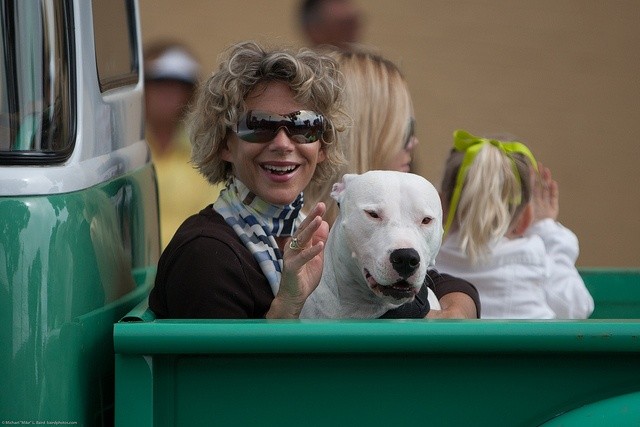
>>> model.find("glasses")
[227,110,324,143]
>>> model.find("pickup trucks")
[0,0,640,427]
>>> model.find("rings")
[289,239,304,250]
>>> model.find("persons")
[300,43,481,318]
[299,0,363,51]
[149,41,355,319]
[141,38,228,255]
[430,128,595,319]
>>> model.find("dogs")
[297,169,445,319]
